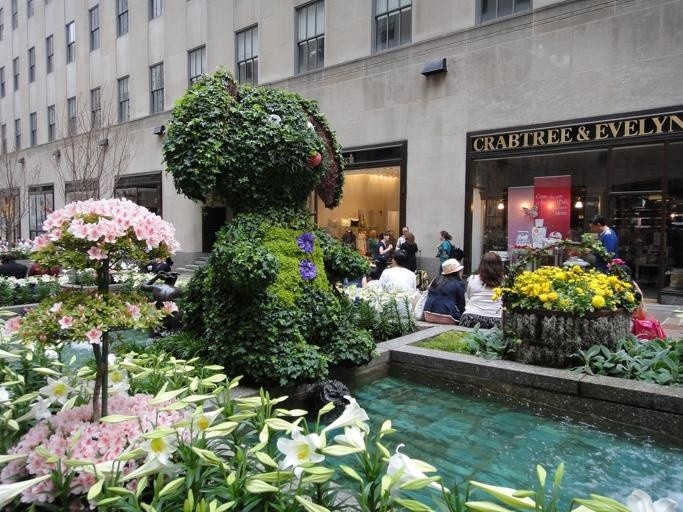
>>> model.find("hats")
[441,258,465,275]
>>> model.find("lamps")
[574,195,584,210]
[422,58,447,76]
[497,197,506,210]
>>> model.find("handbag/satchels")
[414,276,438,321]
[631,313,667,340]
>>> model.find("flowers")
[498,265,637,312]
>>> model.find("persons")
[360,251,421,318]
[423,257,468,326]
[630,279,646,337]
[581,215,619,275]
[331,225,465,290]
[457,252,505,329]
[0,253,28,279]
[140,256,172,273]
[26,261,60,277]
[139,270,182,308]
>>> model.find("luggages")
[415,250,430,291]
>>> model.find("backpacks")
[447,239,465,263]
